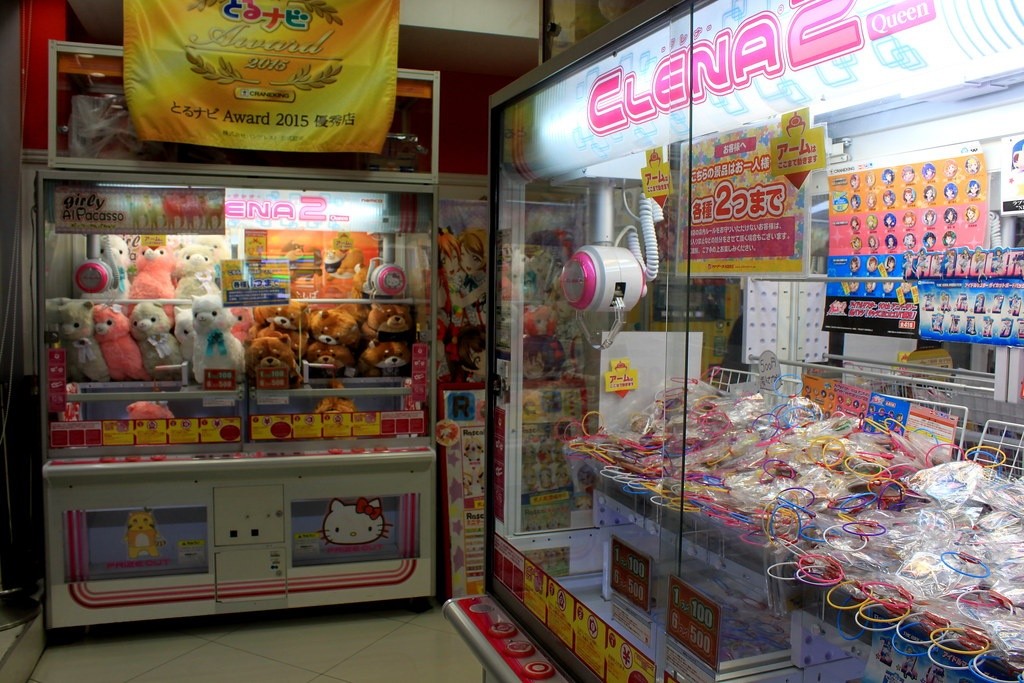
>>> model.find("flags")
[122,1,399,154]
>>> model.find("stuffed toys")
[358,343,411,376]
[350,264,375,324]
[362,303,412,342]
[58,299,109,382]
[312,309,360,345]
[191,293,245,384]
[307,342,355,377]
[245,299,310,388]
[228,308,253,344]
[126,402,175,420]
[128,244,176,328]
[314,382,360,441]
[174,243,221,310]
[130,302,184,381]
[94,304,148,381]
[173,305,194,361]
[81,235,131,300]
